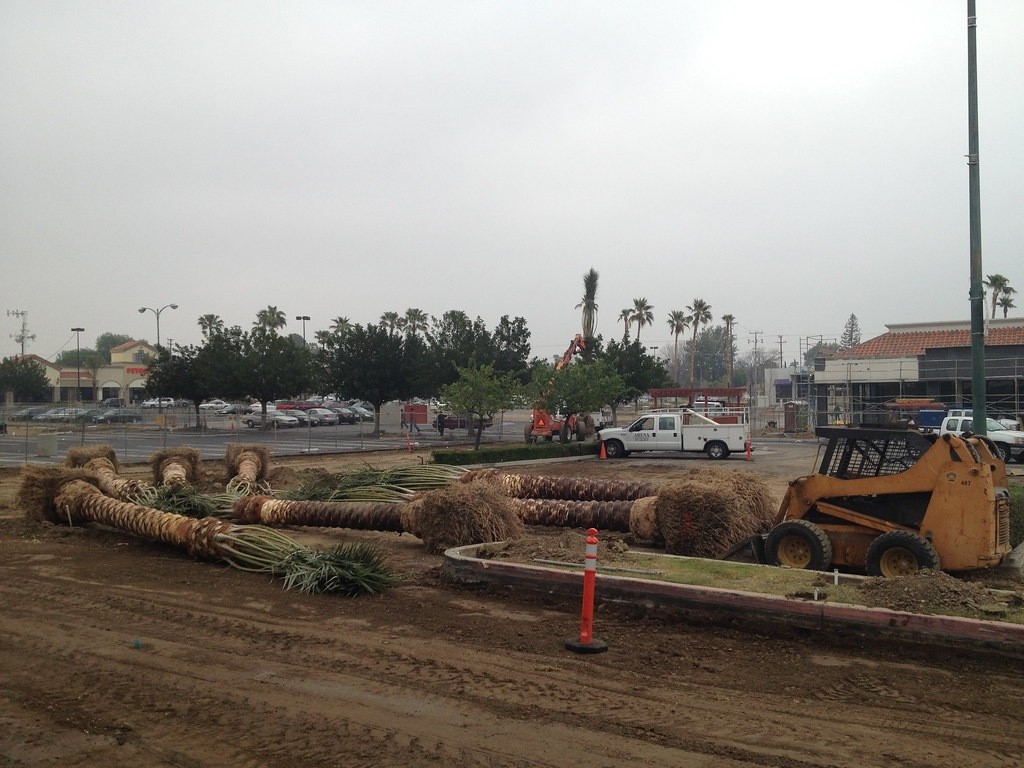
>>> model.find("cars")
[304,407,339,427]
[348,406,375,423]
[241,410,299,430]
[250,401,277,411]
[92,408,142,424]
[12,407,106,424]
[282,410,320,428]
[433,410,492,431]
[330,407,359,425]
[276,395,451,414]
[214,404,252,415]
[199,399,231,410]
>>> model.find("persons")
[438,411,444,436]
[409,408,420,433]
[400,409,408,429]
[907,416,915,424]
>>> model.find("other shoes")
[440,433,444,436]
[409,431,412,432]
[418,430,420,432]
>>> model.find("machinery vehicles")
[523,333,589,445]
[713,419,1013,578]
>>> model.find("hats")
[411,408,414,410]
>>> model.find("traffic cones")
[599,442,608,460]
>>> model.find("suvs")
[98,396,126,408]
[140,397,176,410]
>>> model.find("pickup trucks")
[601,407,755,460]
[908,414,1023,464]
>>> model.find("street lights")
[70,327,84,403]
[138,302,178,430]
[295,315,312,401]
